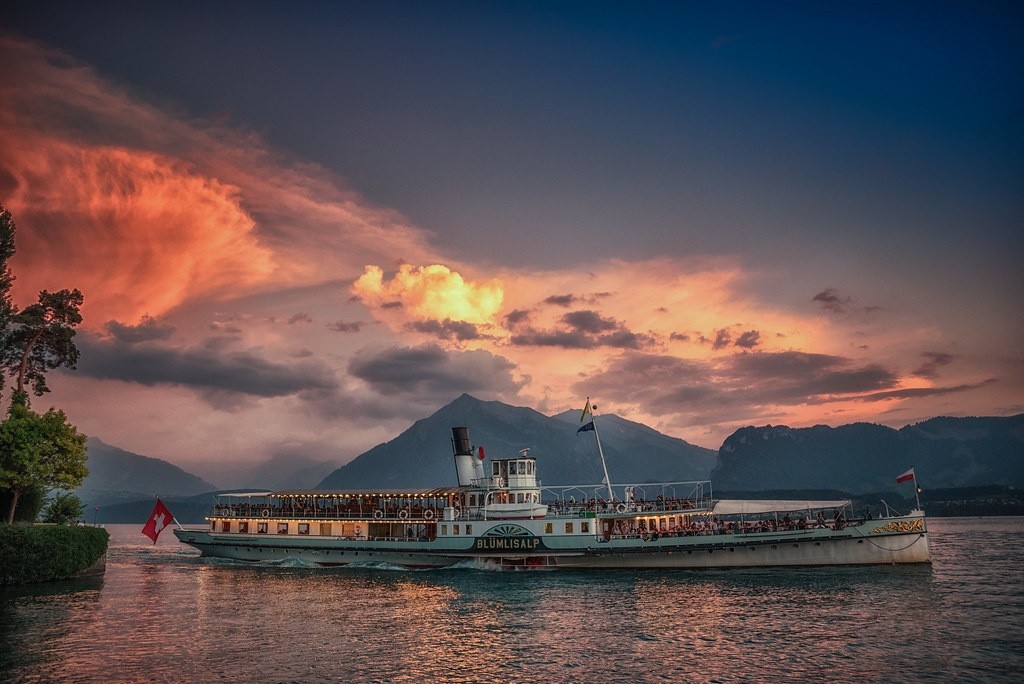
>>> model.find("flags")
[575,400,596,434]
[141,499,174,545]
[897,469,914,484]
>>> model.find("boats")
[171,426,934,574]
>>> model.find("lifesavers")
[262,509,269,518]
[424,510,434,520]
[499,477,504,487]
[616,503,627,514]
[222,508,229,518]
[399,510,408,520]
[375,510,383,520]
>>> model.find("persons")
[510,466,516,474]
[214,496,463,517]
[77,520,86,526]
[525,495,872,541]
